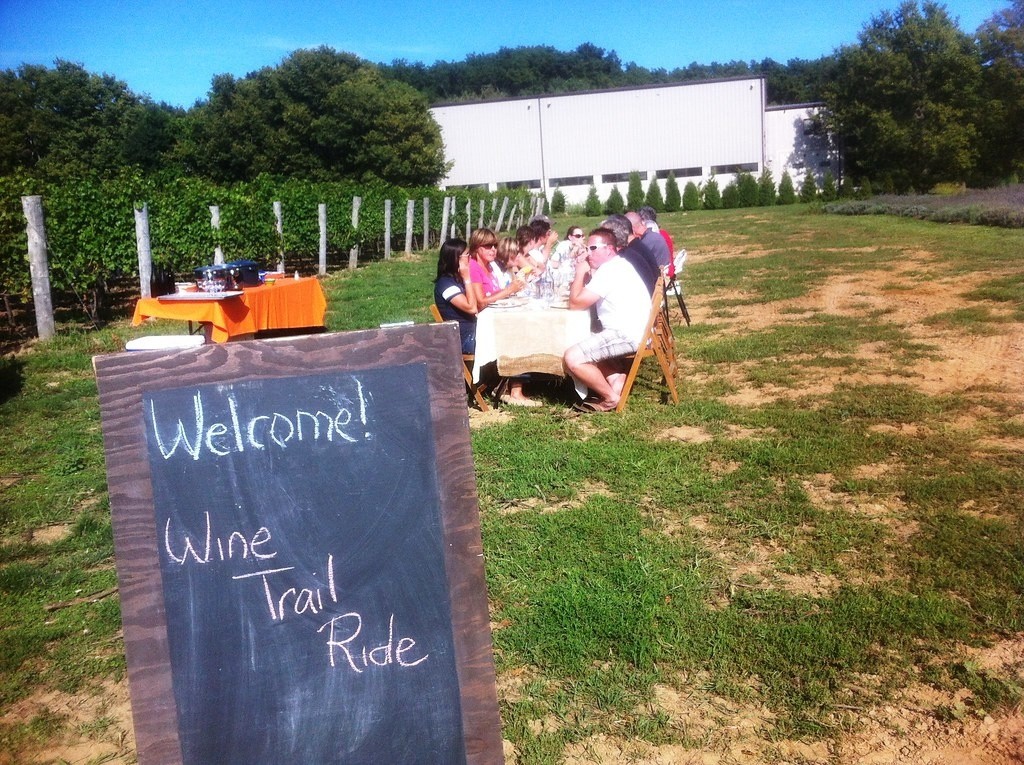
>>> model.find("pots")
[225,260,259,287]
[194,265,222,287]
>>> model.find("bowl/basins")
[265,278,275,287]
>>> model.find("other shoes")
[491,388,511,402]
[507,396,542,407]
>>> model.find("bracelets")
[464,279,472,285]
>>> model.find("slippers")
[573,396,604,413]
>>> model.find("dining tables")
[472,265,603,410]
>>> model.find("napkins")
[496,304,528,314]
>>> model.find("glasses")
[459,253,471,259]
[479,243,498,249]
[572,234,585,238]
[585,244,607,251]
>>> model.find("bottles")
[295,270,299,280]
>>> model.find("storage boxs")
[125,335,205,352]
[205,323,254,344]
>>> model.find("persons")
[434,238,542,408]
[464,228,523,311]
[563,227,653,411]
[490,204,674,301]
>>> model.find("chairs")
[650,278,678,366]
[614,287,677,413]
[429,303,507,412]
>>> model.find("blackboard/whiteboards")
[94,319,509,765]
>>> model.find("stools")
[662,273,692,328]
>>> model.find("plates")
[551,305,569,309]
[488,300,527,308]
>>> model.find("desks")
[131,276,326,344]
